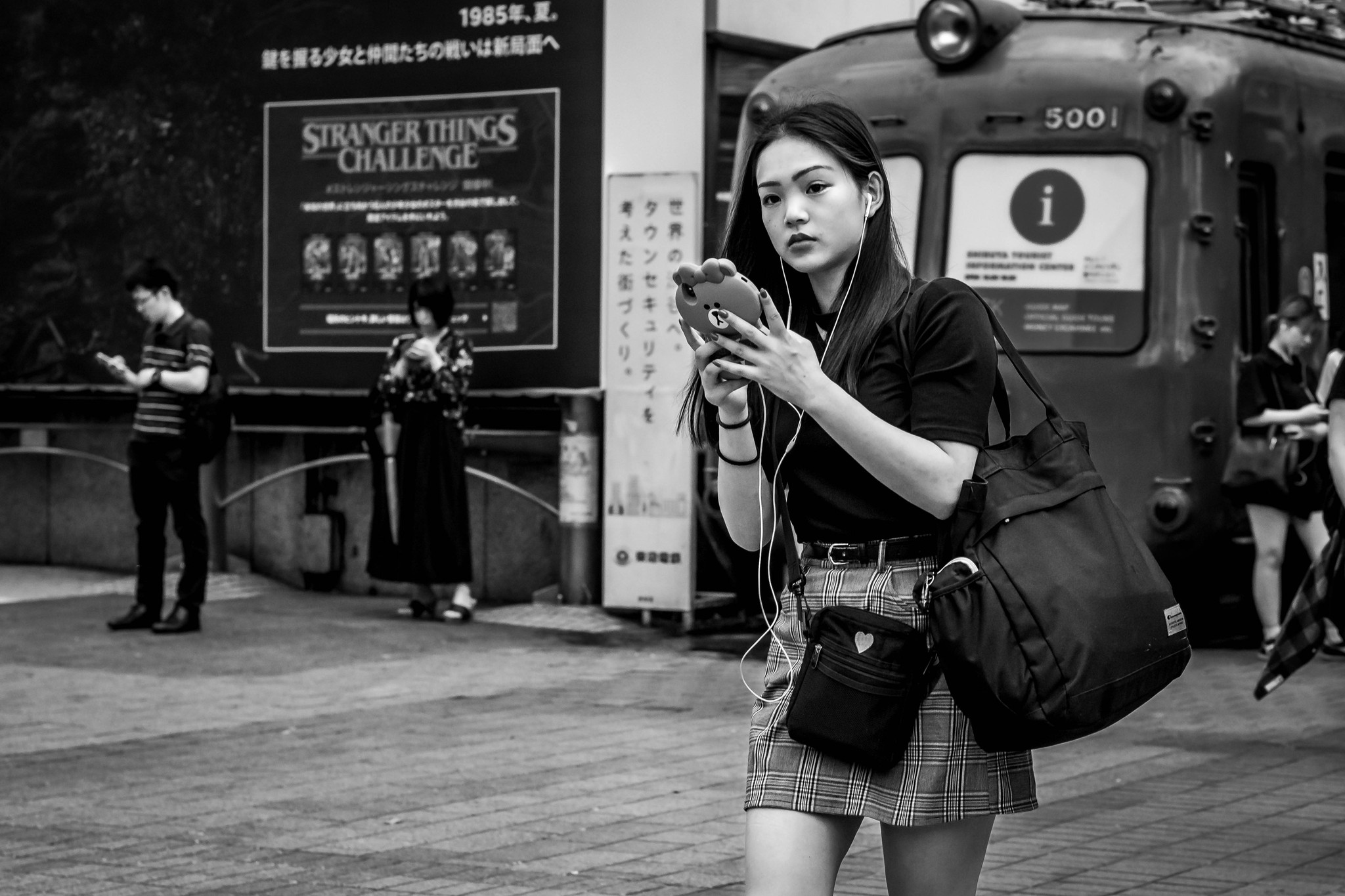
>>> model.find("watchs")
[152,367,162,383]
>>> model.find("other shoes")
[1317,640,1345,660]
[1256,637,1277,661]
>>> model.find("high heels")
[444,598,477,625]
[396,588,441,619]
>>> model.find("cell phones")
[674,257,764,381]
[96,353,127,371]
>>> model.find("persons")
[366,276,475,621]
[1236,294,1345,661]
[675,101,1039,896]
[108,263,213,633]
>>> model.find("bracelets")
[716,440,760,465]
[716,408,751,429]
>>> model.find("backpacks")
[180,315,228,465]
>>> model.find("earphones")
[865,193,873,218]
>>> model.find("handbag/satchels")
[909,420,1192,751]
[785,605,935,775]
[1222,432,1300,501]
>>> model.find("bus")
[726,0,1345,629]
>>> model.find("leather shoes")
[107,604,161,631]
[151,604,203,635]
